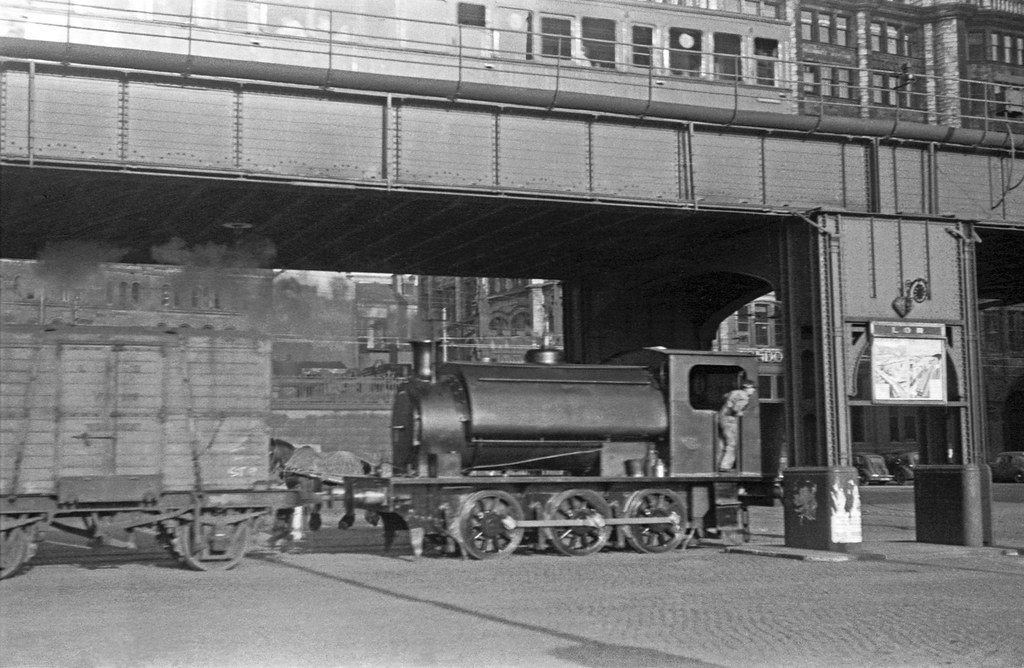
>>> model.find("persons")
[717,380,755,472]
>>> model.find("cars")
[854,451,896,486]
[897,452,919,485]
[990,451,1024,483]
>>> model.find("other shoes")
[718,468,740,475]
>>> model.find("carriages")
[271,434,395,529]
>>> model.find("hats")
[741,383,754,387]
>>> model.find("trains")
[1,277,764,578]
